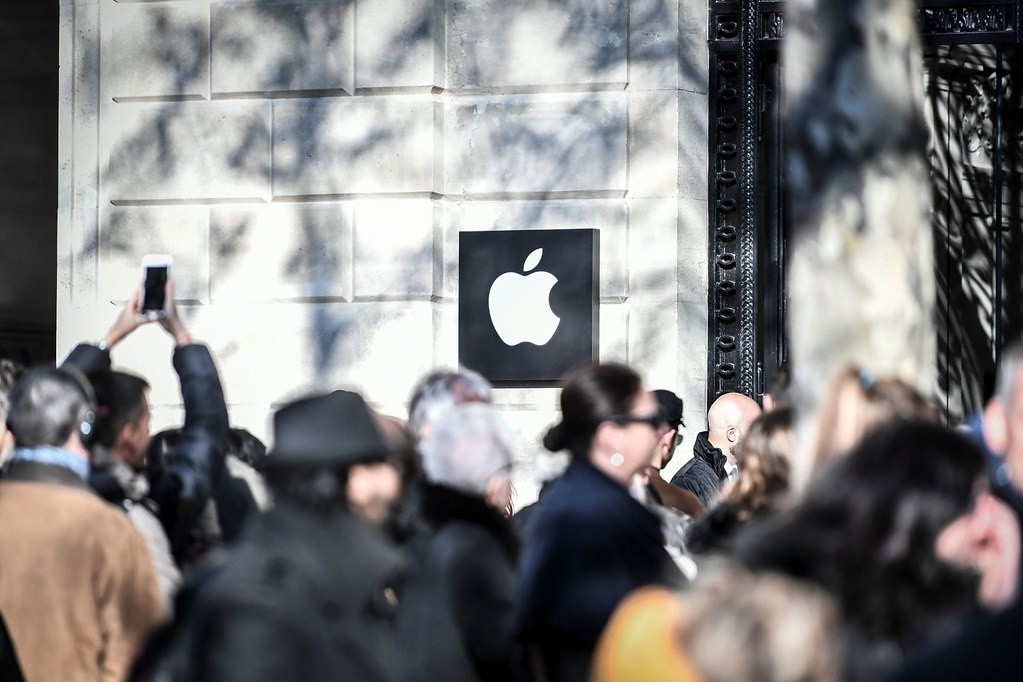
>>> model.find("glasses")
[673,434,684,446]
[610,409,664,427]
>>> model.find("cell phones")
[140,253,172,321]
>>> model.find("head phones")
[7,361,100,449]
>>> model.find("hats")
[254,389,398,464]
[421,396,516,498]
[654,390,688,429]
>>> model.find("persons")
[0,284,1023,682]
